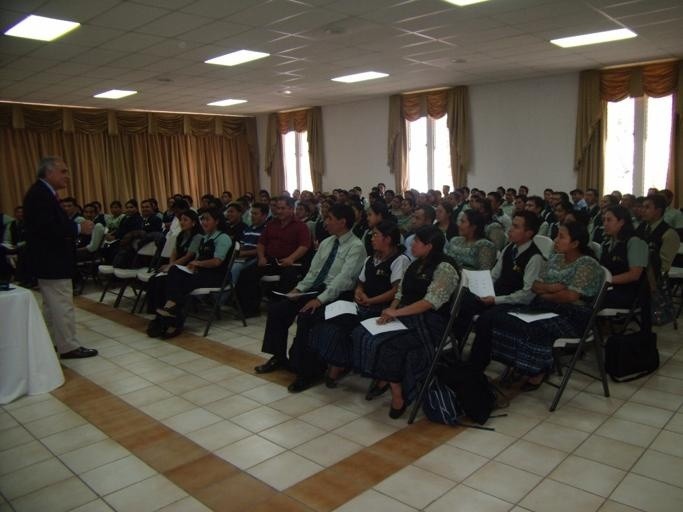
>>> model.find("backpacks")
[423,375,466,426]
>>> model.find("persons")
[8,206,37,289]
[22,158,98,359]
[58,183,683,419]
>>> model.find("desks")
[0,280,64,407]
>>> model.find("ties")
[644,225,652,242]
[312,238,339,290]
[54,192,59,199]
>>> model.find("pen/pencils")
[354,296,362,301]
[278,264,302,267]
[92,214,99,222]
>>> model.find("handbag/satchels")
[195,250,233,289]
[605,316,660,383]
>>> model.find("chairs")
[75,226,682,431]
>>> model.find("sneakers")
[158,302,179,318]
[219,299,260,321]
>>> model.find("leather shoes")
[325,374,338,389]
[521,374,551,390]
[53,344,96,358]
[255,358,284,372]
[366,384,388,400]
[288,376,314,391]
[389,400,407,419]
